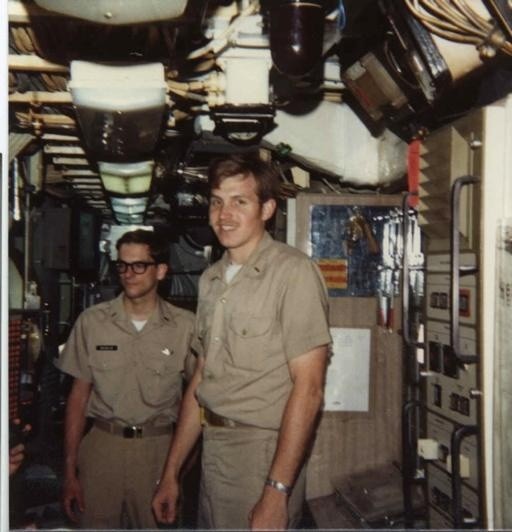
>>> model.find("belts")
[92,416,175,440]
[203,405,255,430]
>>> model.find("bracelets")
[263,478,294,498]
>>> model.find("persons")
[51,229,199,529]
[150,152,332,530]
[10,418,32,478]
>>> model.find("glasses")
[111,258,161,275]
